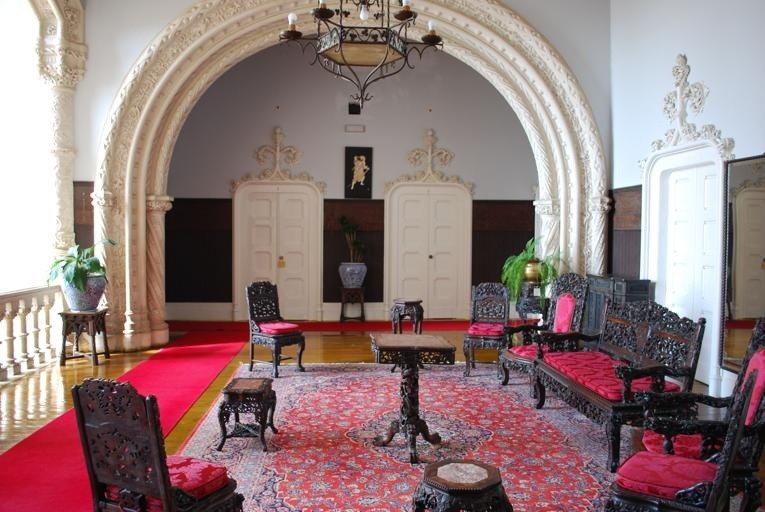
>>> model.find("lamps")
[278,0,445,111]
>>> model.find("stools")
[215,377,278,453]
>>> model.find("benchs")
[531,295,706,474]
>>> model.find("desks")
[58,306,112,367]
[340,287,365,322]
[367,333,457,463]
[388,296,428,373]
[412,456,514,512]
[515,293,550,322]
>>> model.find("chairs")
[463,282,511,379]
[71,377,244,511]
[605,365,758,512]
[498,271,591,398]
[633,315,764,511]
[245,280,307,378]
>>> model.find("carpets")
[171,359,648,512]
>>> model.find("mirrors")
[716,153,765,376]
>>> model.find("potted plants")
[338,230,367,288]
[44,238,118,311]
[500,235,566,309]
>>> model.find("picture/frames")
[344,145,374,199]
